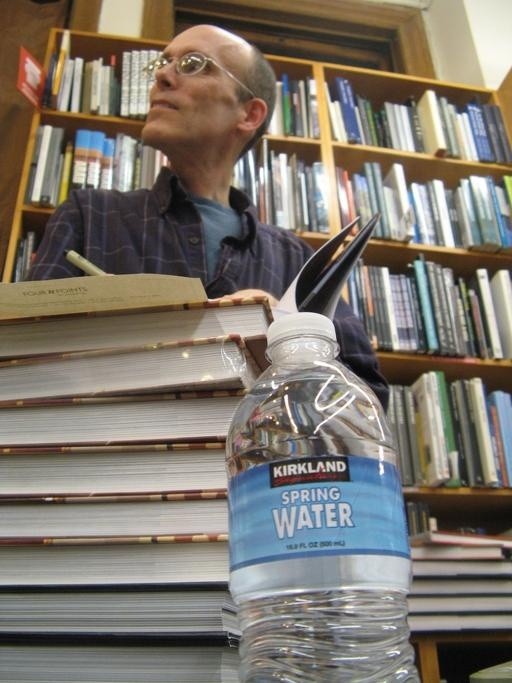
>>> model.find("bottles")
[221,309,420,683]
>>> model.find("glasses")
[141,51,256,99]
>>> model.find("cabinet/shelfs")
[2,23,512,681]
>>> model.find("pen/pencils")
[62,248,109,276]
